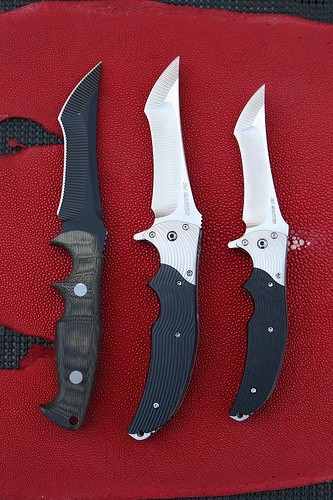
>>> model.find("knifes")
[128,54,202,445]
[37,56,106,433]
[223,83,292,422]
